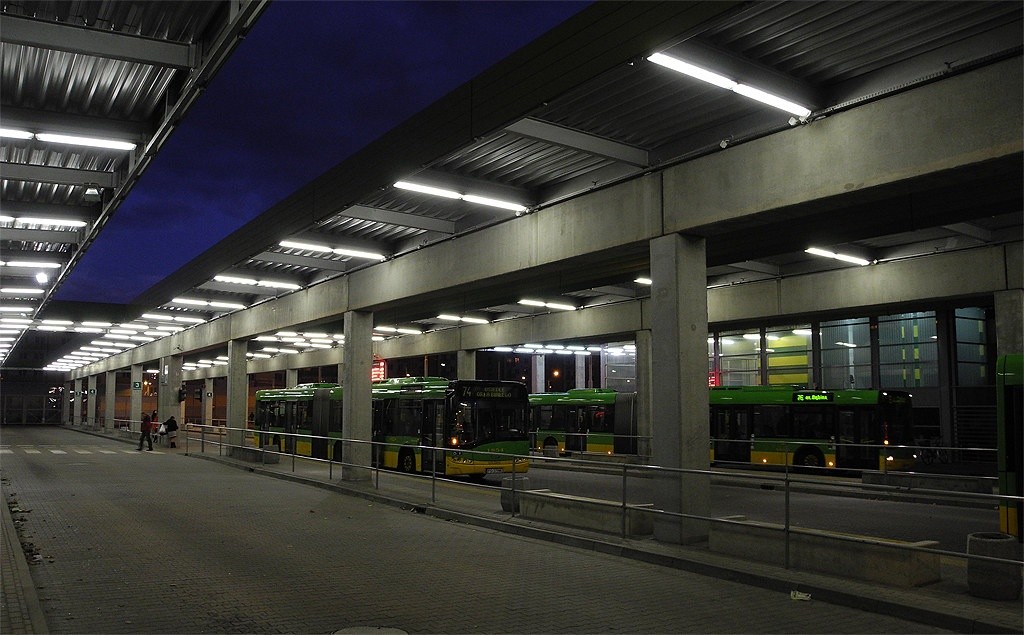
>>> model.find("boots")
[170,442,173,448]
[173,442,176,448]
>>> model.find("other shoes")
[146,448,153,451]
[136,448,141,450]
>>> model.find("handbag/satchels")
[159,424,165,433]
[160,429,168,434]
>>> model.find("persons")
[162,415,179,448]
[150,409,159,443]
[135,411,154,451]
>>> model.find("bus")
[527,387,618,464]
[709,383,917,478]
[254,376,532,480]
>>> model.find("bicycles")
[919,434,948,466]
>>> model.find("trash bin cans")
[967,531,1020,599]
[264,445,280,464]
[502,476,530,512]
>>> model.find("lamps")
[645,50,813,121]
[0,125,577,371]
